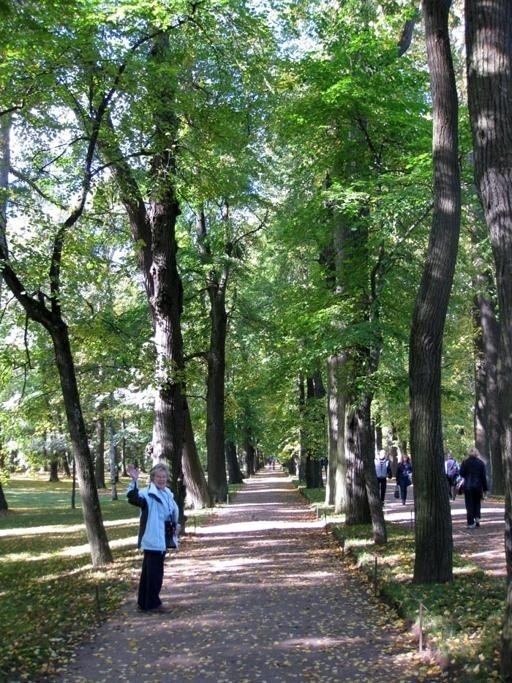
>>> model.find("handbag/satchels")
[455,478,465,496]
[394,491,399,498]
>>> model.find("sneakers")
[138,604,173,614]
[465,523,475,529]
[475,517,480,526]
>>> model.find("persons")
[125,461,180,612]
[459,445,490,529]
[396,454,413,506]
[373,449,393,505]
[443,454,459,501]
